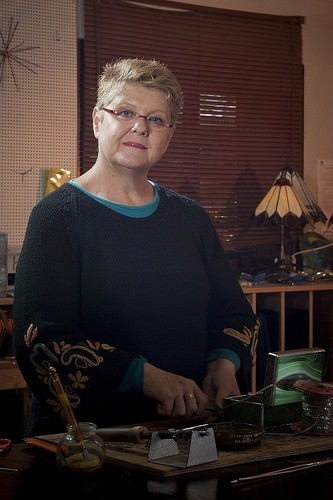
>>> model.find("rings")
[184,393,195,400]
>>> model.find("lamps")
[253,168,328,283]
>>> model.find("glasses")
[102,105,173,131]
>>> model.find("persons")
[9,55,256,443]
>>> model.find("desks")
[239,277,333,391]
[24,419,333,499]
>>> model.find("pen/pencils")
[48,367,88,461]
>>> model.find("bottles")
[55,422,106,472]
